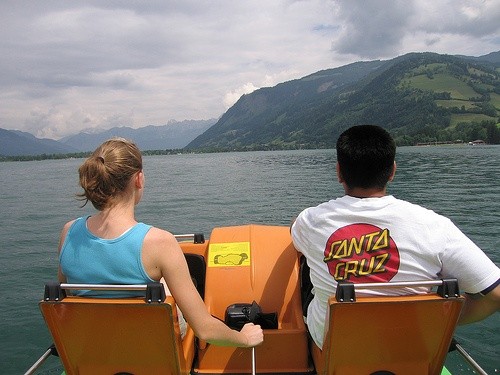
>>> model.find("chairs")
[38,281,196,375]
[310,279,467,375]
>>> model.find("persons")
[288,124,500,375]
[56,137,263,365]
